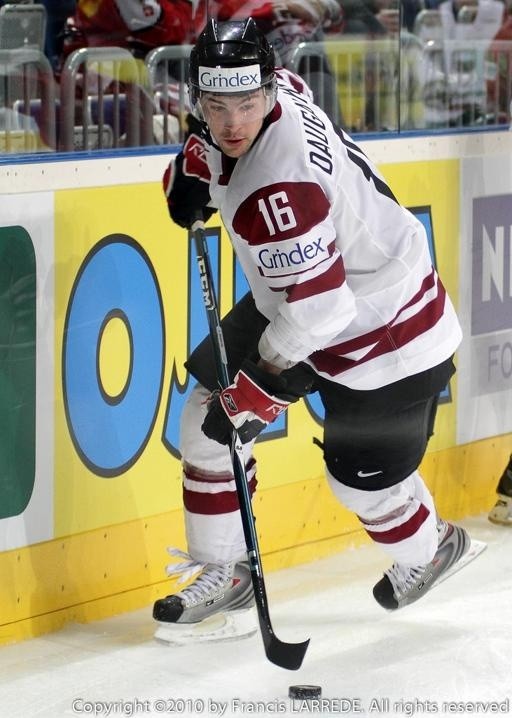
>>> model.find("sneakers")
[372,515,470,609]
[485,494,512,527]
[152,556,256,625]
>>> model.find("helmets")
[186,15,277,106]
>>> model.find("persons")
[1,1,511,159]
[148,15,468,625]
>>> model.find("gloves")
[160,111,222,234]
[199,352,318,451]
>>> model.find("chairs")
[0,0,510,155]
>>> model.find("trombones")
[190,208,310,670]
